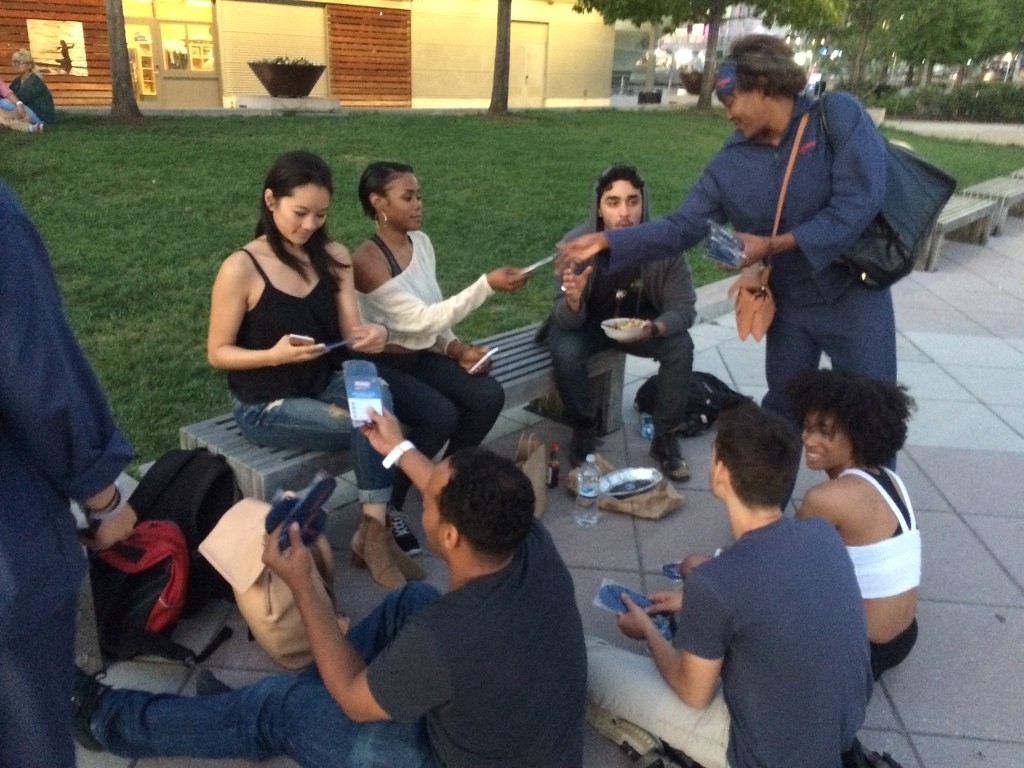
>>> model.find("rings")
[561,286,566,291]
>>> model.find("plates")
[601,466,663,498]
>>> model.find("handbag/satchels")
[568,452,685,520]
[816,96,957,290]
[636,372,743,436]
[509,431,547,519]
[728,259,775,342]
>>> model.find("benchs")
[914,194,996,272]
[1012,167,1024,179]
[961,176,1024,236]
[179,320,626,503]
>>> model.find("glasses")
[13,60,28,65]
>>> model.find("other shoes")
[567,420,599,469]
[351,515,405,593]
[30,122,43,133]
[584,705,668,762]
[649,439,691,480]
[382,530,426,581]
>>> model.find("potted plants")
[249,56,326,98]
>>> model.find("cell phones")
[467,347,498,374]
[288,334,315,347]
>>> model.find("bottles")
[572,455,601,523]
[547,444,559,487]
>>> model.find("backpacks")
[90,446,249,665]
[199,498,347,674]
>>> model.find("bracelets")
[16,101,23,105]
[88,487,124,520]
[381,440,416,469]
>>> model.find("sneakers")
[194,670,230,701]
[60,663,117,754]
[386,505,422,554]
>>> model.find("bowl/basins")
[600,318,648,342]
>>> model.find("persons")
[207,151,429,590]
[584,404,874,768]
[0,49,57,132]
[534,163,698,478]
[0,180,138,768]
[353,161,530,556]
[72,407,588,768]
[786,366,921,682]
[559,34,898,512]
